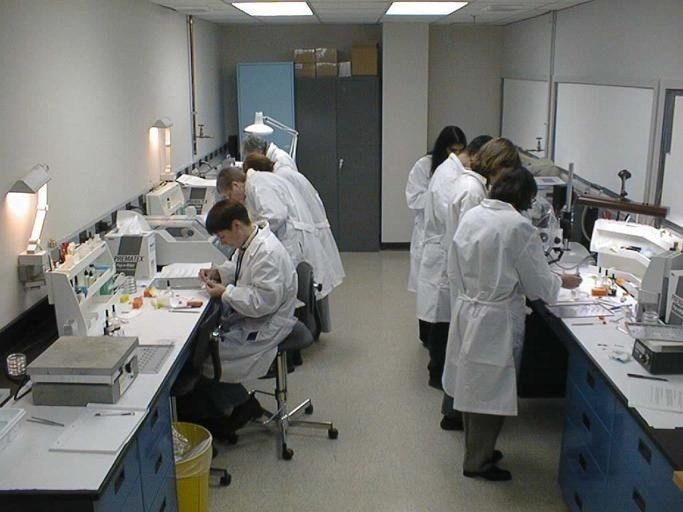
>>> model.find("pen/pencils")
[627,373,668,381]
[95,412,135,416]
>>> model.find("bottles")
[166,279,173,298]
[101,304,122,337]
[72,263,96,303]
[592,266,617,297]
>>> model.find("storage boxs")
[293,48,315,63]
[294,63,315,78]
[350,40,380,75]
[316,64,338,78]
[315,48,338,64]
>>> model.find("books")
[48,402,149,453]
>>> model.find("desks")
[0,286,211,512]
[522,239,683,511]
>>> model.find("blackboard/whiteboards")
[654,88,683,236]
[499,77,549,159]
[551,81,661,206]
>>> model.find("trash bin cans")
[171,422,213,512]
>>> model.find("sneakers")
[463,449,511,481]
[222,396,264,432]
[440,415,463,430]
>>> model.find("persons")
[441,164,582,481]
[406,126,467,347]
[243,152,345,333]
[242,133,297,170]
[416,135,493,390]
[200,198,299,440]
[440,137,522,431]
[217,167,338,374]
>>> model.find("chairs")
[243,261,338,461]
[166,304,233,487]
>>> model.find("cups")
[641,311,660,325]
[124,276,137,294]
[7,354,27,376]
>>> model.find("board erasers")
[591,183,604,190]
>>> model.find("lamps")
[6,161,51,253]
[242,110,300,160]
[151,118,177,181]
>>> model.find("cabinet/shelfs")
[295,75,381,252]
[234,61,294,160]
